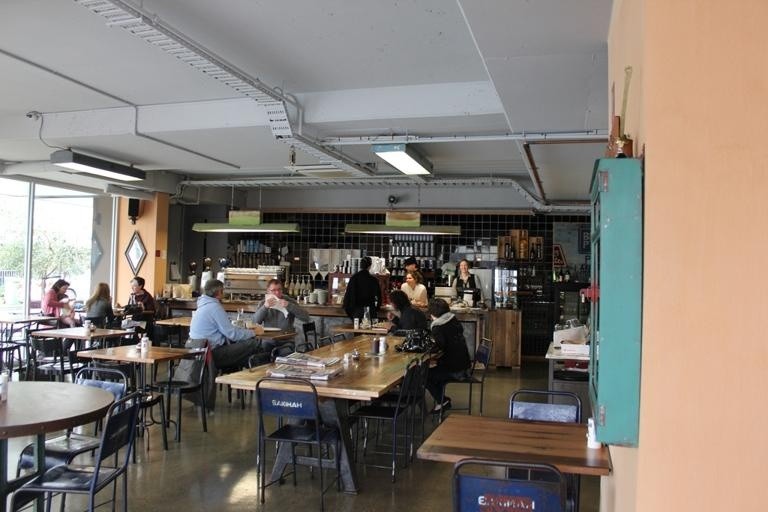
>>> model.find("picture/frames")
[91,231,103,274]
[125,232,148,276]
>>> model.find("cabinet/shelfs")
[588,157,643,446]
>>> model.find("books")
[265,352,343,381]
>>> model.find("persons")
[114,276,156,332]
[343,255,484,337]
[41,279,83,355]
[422,298,472,413]
[189,279,265,369]
[252,279,310,364]
[57,294,80,327]
[87,282,119,328]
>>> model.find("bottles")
[510,243,515,259]
[140,333,148,352]
[518,264,536,277]
[351,348,361,359]
[536,239,541,258]
[558,268,570,282]
[392,234,435,256]
[530,242,535,260]
[288,274,312,299]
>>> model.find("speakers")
[128,199,139,216]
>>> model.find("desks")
[545,342,599,424]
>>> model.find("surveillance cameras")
[388,195,398,204]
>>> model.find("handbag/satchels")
[124,304,143,315]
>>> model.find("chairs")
[451,458,567,511]
[438,337,493,426]
[506,390,581,511]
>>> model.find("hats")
[404,256,417,264]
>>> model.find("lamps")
[191,185,301,234]
[370,143,433,175]
[51,149,146,183]
[344,185,462,237]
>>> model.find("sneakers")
[431,397,451,414]
[395,329,435,353]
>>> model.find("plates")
[263,327,282,332]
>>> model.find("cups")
[354,317,359,329]
[256,321,264,328]
[85,320,91,336]
[297,296,299,303]
[371,336,386,353]
[308,289,328,306]
[372,319,378,326]
[304,297,308,304]
[588,418,602,450]
[0,373,8,403]
